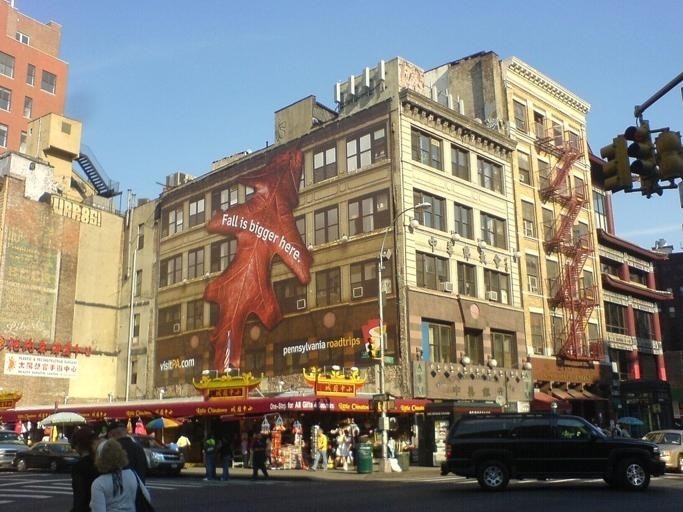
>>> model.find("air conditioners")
[439,282,453,292]
[485,290,497,301]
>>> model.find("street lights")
[378,202,432,473]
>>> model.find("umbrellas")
[127,415,178,445]
[39,412,86,438]
[617,417,644,434]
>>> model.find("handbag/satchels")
[130,469,155,512]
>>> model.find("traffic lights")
[367,335,376,361]
[599,134,632,195]
[654,129,682,180]
[625,120,662,198]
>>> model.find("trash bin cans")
[395,451,410,471]
[355,443,374,474]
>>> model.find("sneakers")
[203,475,229,481]
[248,475,270,481]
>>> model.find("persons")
[68,421,157,512]
[218,434,233,480]
[177,433,192,456]
[335,429,352,472]
[201,434,219,480]
[250,432,270,482]
[388,436,396,457]
[310,427,328,471]
[619,426,631,438]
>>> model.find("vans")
[0,431,31,471]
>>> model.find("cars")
[440,410,664,493]
[641,428,683,474]
[97,434,186,476]
[12,440,81,473]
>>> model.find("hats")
[104,422,127,439]
